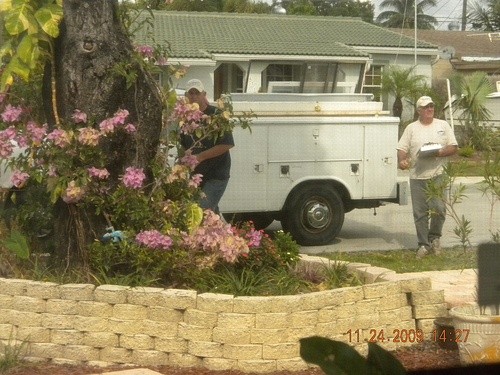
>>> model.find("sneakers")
[416,246,429,258]
[431,238,441,255]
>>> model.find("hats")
[185,79,204,93]
[417,96,435,107]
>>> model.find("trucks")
[162,86,409,246]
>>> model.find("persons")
[396,95,458,258]
[177,79,236,224]
